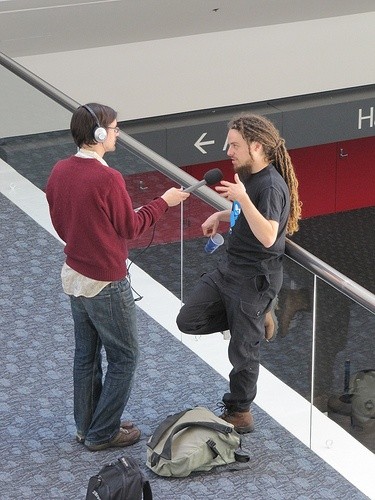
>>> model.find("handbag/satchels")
[86,457,152,500]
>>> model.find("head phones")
[78,105,108,142]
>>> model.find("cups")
[203,232,224,254]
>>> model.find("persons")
[45,103,191,451]
[176,114,302,433]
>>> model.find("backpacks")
[146,406,250,477]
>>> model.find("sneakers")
[213,402,253,434]
[265,294,279,343]
[76,419,133,443]
[86,426,141,451]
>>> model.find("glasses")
[105,126,119,134]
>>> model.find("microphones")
[183,168,224,192]
